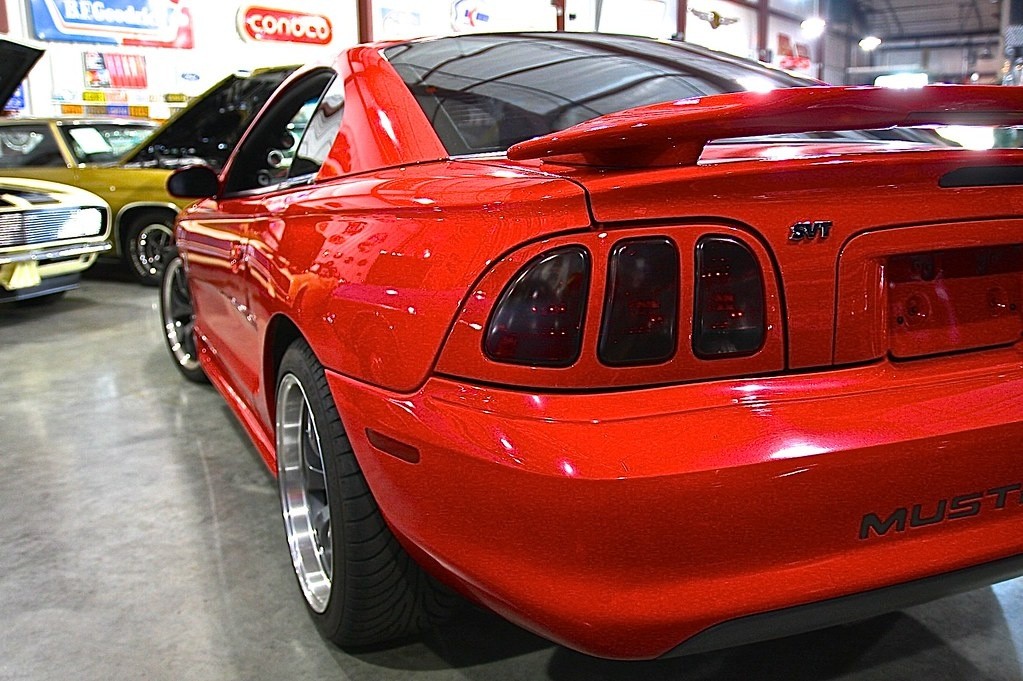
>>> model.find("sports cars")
[160,31,1022,661]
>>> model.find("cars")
[0,39,303,308]
